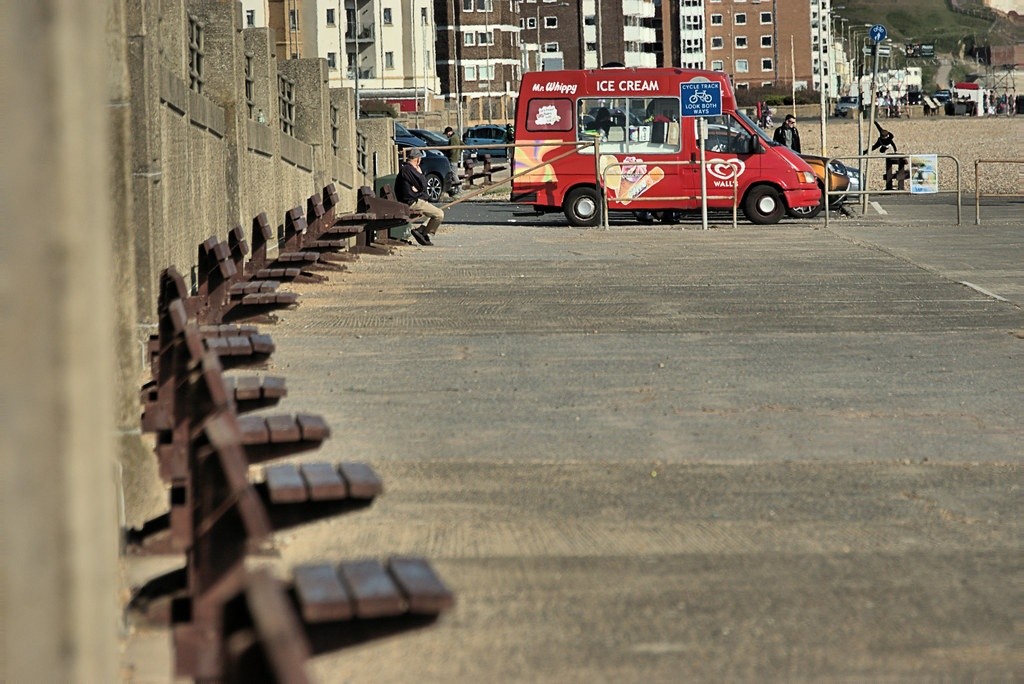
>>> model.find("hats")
[409,148,422,156]
[443,127,452,134]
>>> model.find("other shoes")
[426,240,433,245]
[411,230,427,245]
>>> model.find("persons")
[643,101,674,127]
[859,87,1016,119]
[773,115,800,154]
[761,100,770,130]
[501,123,514,163]
[863,120,897,155]
[441,126,463,194]
[393,149,443,246]
[585,107,617,138]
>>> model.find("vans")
[511,67,822,225]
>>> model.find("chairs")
[120,415,457,684]
[144,264,259,357]
[327,184,376,265]
[375,183,423,247]
[350,185,412,256]
[288,206,347,270]
[311,193,364,273]
[123,353,332,558]
[141,300,277,397]
[139,325,289,431]
[255,212,329,283]
[234,226,300,280]
[215,240,282,295]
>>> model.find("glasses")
[788,120,795,124]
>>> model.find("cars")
[844,166,866,200]
[406,130,471,160]
[933,90,951,103]
[394,122,453,203]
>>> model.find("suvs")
[834,95,862,117]
[696,124,851,219]
[463,124,507,157]
[900,91,928,105]
[588,105,646,125]
